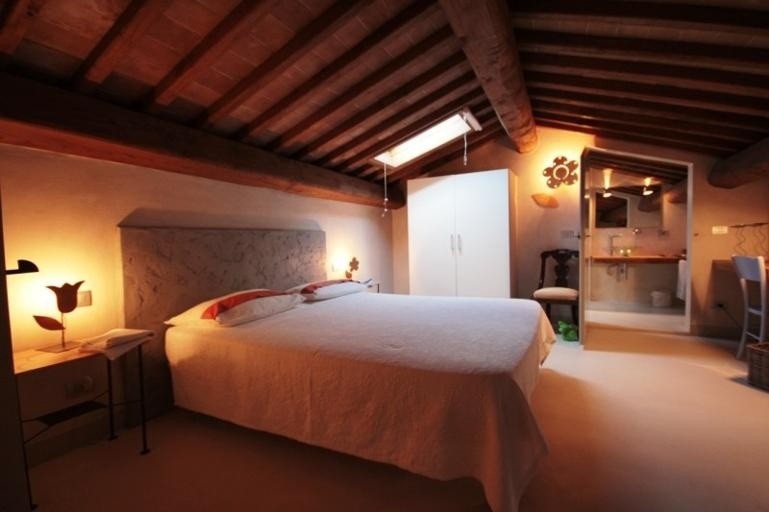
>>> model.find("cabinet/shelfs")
[404,168,517,299]
[14,327,153,453]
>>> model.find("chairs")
[529,247,579,329]
[731,254,769,360]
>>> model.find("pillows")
[163,279,375,329]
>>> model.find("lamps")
[30,278,90,355]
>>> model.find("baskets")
[746,342,769,390]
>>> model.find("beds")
[111,228,557,511]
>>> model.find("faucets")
[607,234,623,255]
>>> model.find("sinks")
[605,247,637,256]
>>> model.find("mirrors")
[593,183,664,228]
